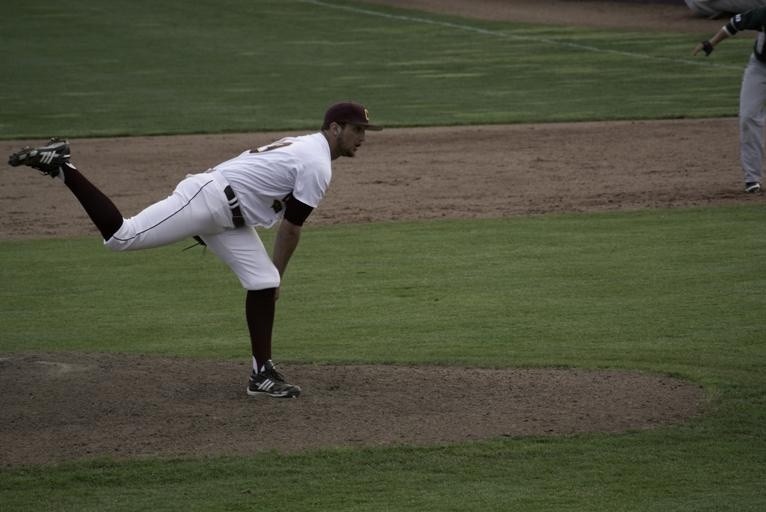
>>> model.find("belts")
[225,185,246,226]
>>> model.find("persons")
[7,101,383,398]
[694,4,766,193]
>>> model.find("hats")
[324,103,383,130]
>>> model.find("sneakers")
[745,182,761,192]
[247,360,301,397]
[7,137,71,178]
